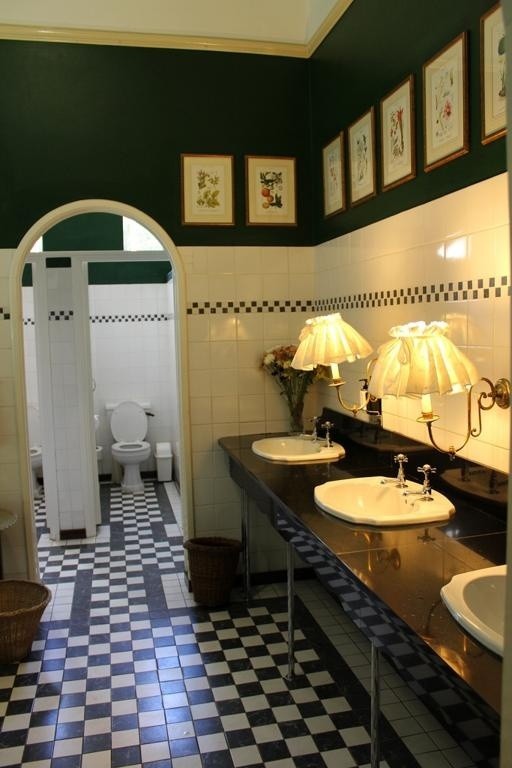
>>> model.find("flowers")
[259,343,329,428]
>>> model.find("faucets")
[400,464,437,496]
[312,421,334,447]
[381,453,408,488]
[299,416,322,440]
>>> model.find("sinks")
[440,564,508,659]
[251,434,345,462]
[313,476,456,528]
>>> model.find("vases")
[285,401,307,436]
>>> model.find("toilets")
[30,447,43,495]
[105,400,155,490]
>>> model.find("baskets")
[0,578,52,664]
[183,535,241,606]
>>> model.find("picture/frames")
[478,2,508,147]
[421,28,473,174]
[179,152,236,229]
[245,154,298,228]
[346,103,379,208]
[321,131,346,221]
[379,73,418,194]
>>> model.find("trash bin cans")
[155,442,173,482]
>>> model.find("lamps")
[419,591,483,687]
[289,309,379,415]
[345,526,401,588]
[367,319,511,455]
